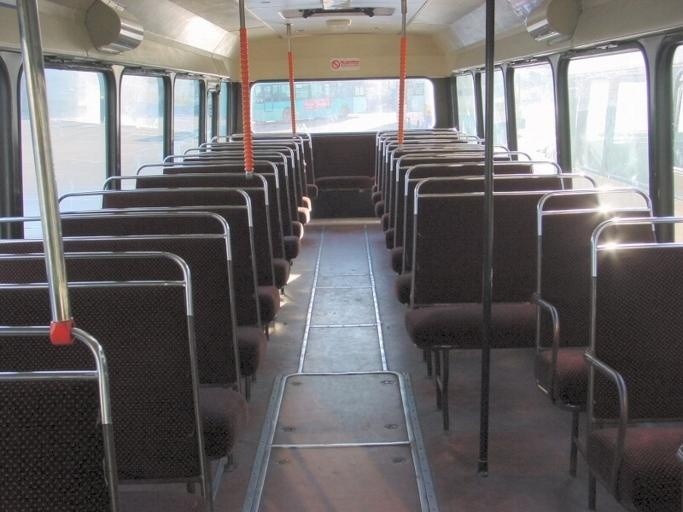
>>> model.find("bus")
[195,79,430,125]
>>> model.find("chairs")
[532,188,657,477]
[281,133,318,288]
[585,216,683,512]
[315,133,371,188]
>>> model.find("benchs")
[368,128,598,433]
[0,134,281,512]
